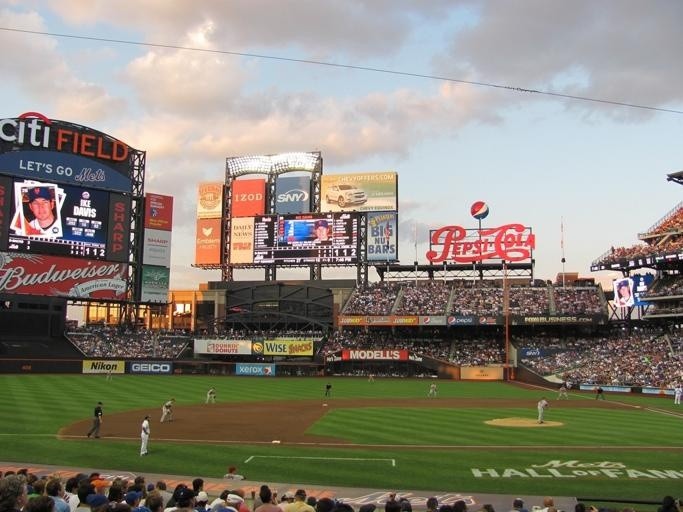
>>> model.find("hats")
[544,496,553,506]
[619,281,628,287]
[27,187,51,202]
[196,492,207,503]
[126,491,144,503]
[315,220,328,229]
[514,498,523,507]
[91,480,104,487]
[227,493,243,504]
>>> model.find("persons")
[536,396,551,424]
[24,185,60,236]
[0,462,683,512]
[137,413,152,457]
[63,203,683,404]
[159,396,175,423]
[86,400,103,439]
